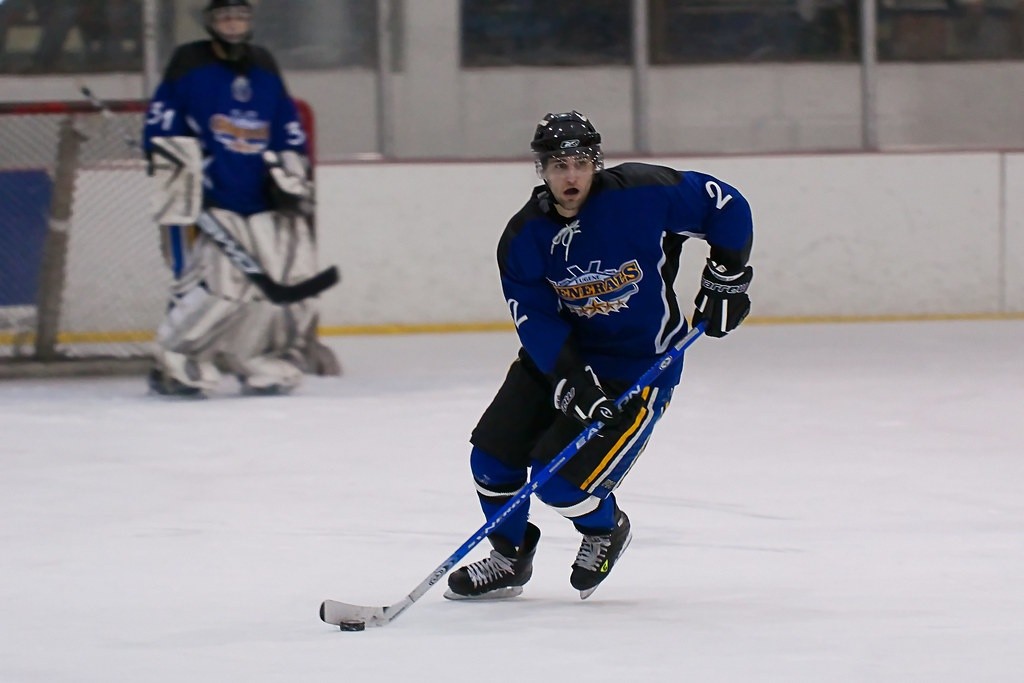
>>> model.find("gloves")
[692,256,753,338]
[551,367,619,437]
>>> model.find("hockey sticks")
[319,318,708,628]
[79,83,341,305]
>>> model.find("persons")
[0,0,174,72]
[141,0,307,399]
[443,110,754,600]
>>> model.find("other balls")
[340,619,365,631]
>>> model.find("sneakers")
[570,492,632,600]
[443,521,541,600]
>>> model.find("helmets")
[531,111,604,178]
[204,0,251,13]
